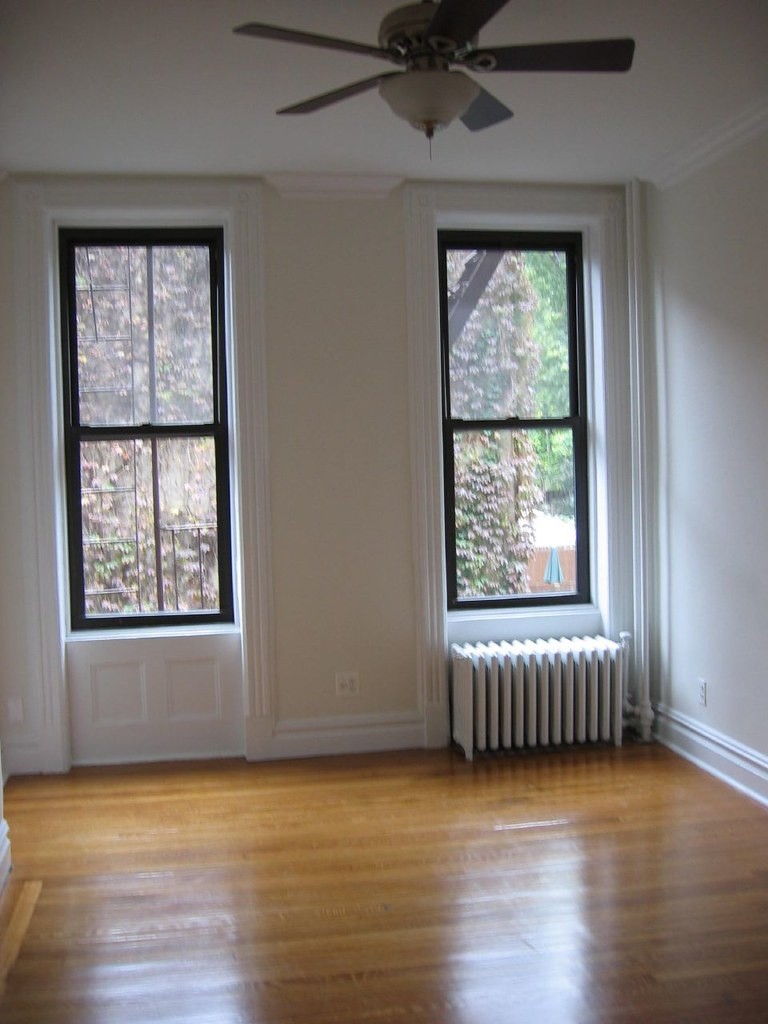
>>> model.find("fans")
[232,0,635,161]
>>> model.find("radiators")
[453,628,632,761]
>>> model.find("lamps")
[378,70,473,160]
